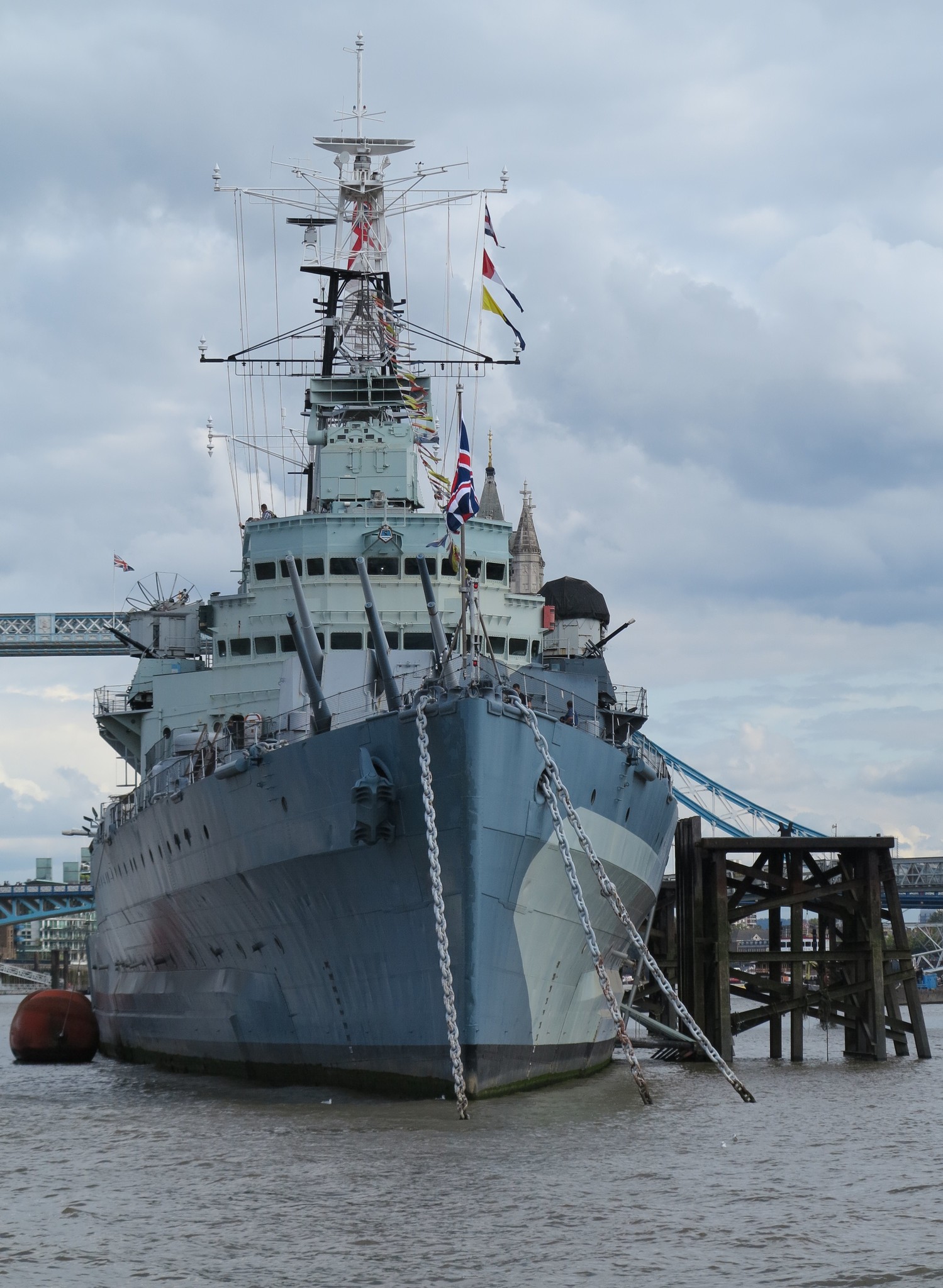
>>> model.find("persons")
[257,504,278,521]
[508,684,534,708]
[559,700,580,729]
[238,517,253,530]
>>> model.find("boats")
[58,27,679,1105]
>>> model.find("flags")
[483,248,523,313]
[114,554,135,572]
[485,203,506,248]
[445,415,480,535]
[479,283,527,351]
